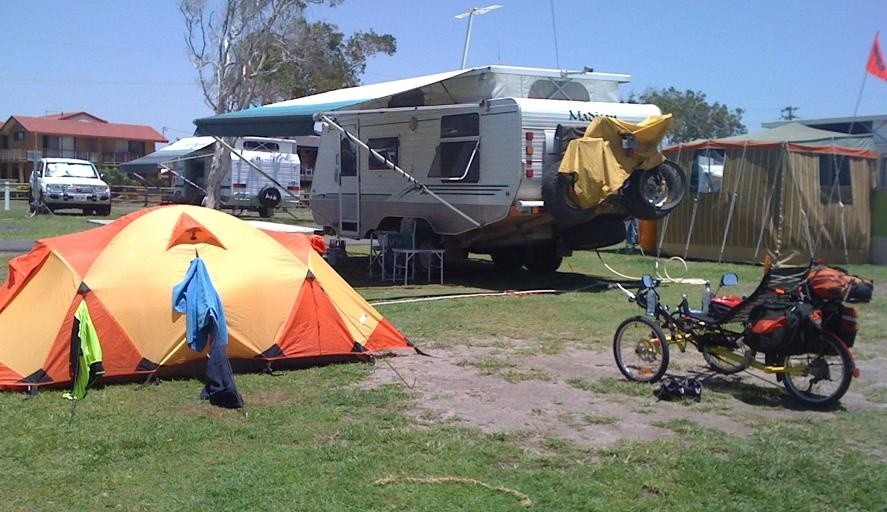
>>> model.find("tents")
[1,202,410,396]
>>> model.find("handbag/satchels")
[796,268,873,355]
[743,298,825,355]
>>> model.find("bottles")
[701,283,711,313]
[645,287,656,316]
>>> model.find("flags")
[867,35,887,81]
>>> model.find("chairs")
[367,218,419,281]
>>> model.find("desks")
[390,247,447,285]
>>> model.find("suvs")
[28,154,114,217]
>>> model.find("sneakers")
[653,378,701,403]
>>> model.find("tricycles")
[587,256,864,408]
